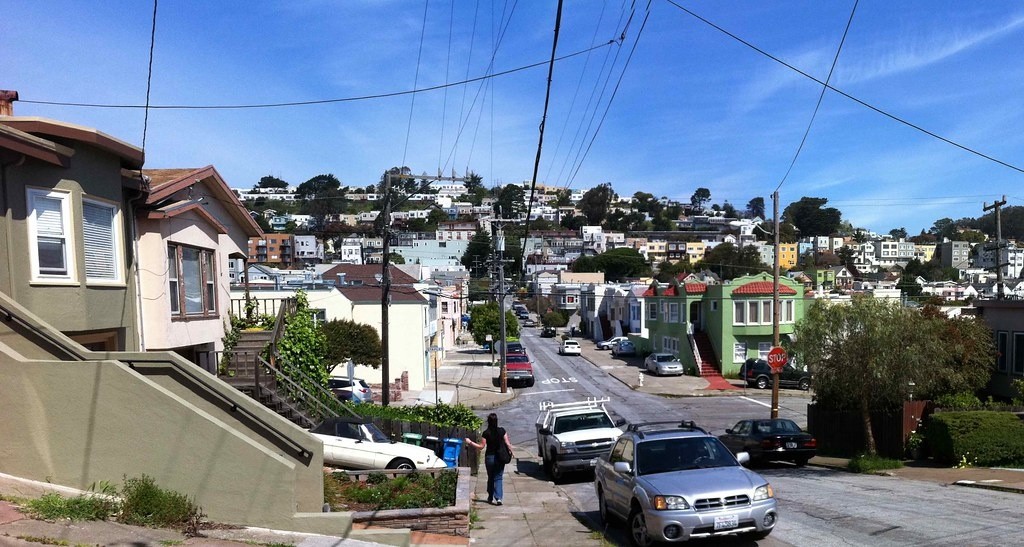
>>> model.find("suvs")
[499,353,534,387]
[738,358,812,391]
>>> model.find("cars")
[612,341,636,357]
[516,307,525,315]
[543,326,556,336]
[559,340,581,355]
[597,336,628,350]
[308,417,447,480]
[506,337,519,343]
[719,419,817,470]
[644,352,684,377]
[328,375,373,405]
[507,341,522,353]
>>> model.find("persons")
[464,413,515,506]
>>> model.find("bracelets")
[469,441,472,445]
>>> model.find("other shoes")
[487,498,492,504]
[497,500,503,505]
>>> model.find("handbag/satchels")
[498,427,511,465]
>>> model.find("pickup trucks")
[594,421,778,547]
[524,319,534,326]
[535,397,625,480]
[513,301,523,309]
[520,310,529,318]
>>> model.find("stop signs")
[767,348,787,368]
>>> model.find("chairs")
[556,419,571,433]
[647,450,678,466]
